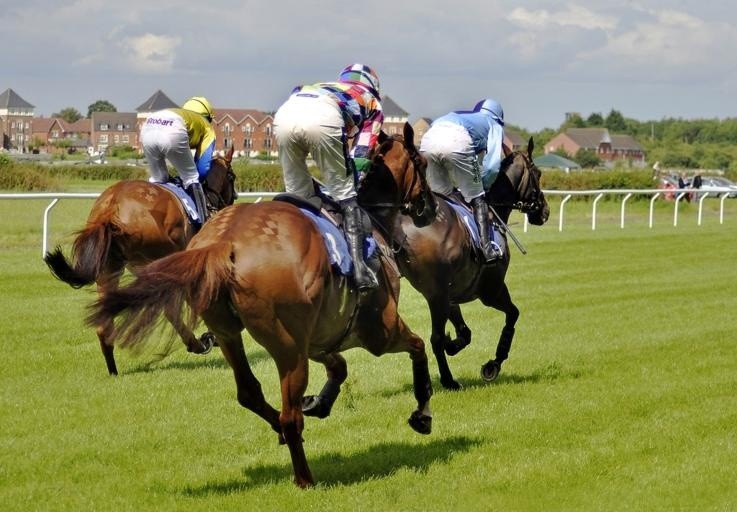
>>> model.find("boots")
[340,197,377,289]
[471,195,501,263]
[187,182,210,227]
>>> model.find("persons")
[689,169,702,203]
[98,150,104,165]
[416,97,507,265]
[271,62,386,291]
[678,171,690,203]
[138,97,217,227]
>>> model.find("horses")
[81,121,440,491]
[390,133,552,393]
[40,141,239,376]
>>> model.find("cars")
[656,174,737,200]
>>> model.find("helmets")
[182,96,211,120]
[339,63,380,97]
[474,99,504,125]
